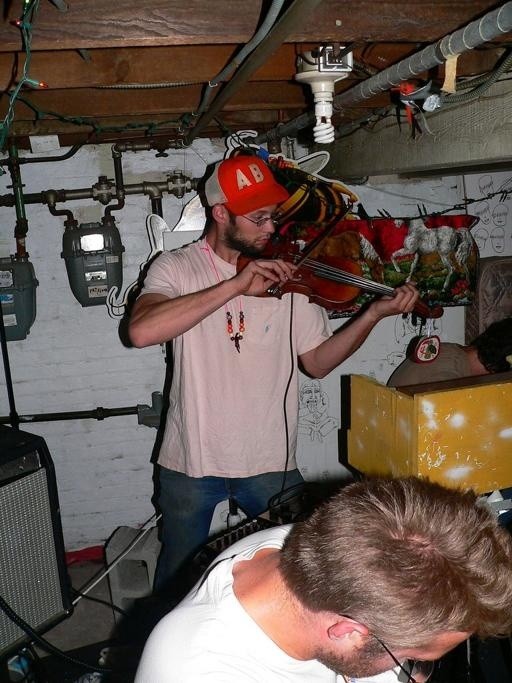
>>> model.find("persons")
[126,152,421,596]
[135,471,512,679]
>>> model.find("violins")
[236,243,444,325]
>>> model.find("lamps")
[291,45,353,146]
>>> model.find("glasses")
[344,616,435,683]
[242,213,281,226]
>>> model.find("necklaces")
[203,232,251,355]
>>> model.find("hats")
[204,155,290,215]
